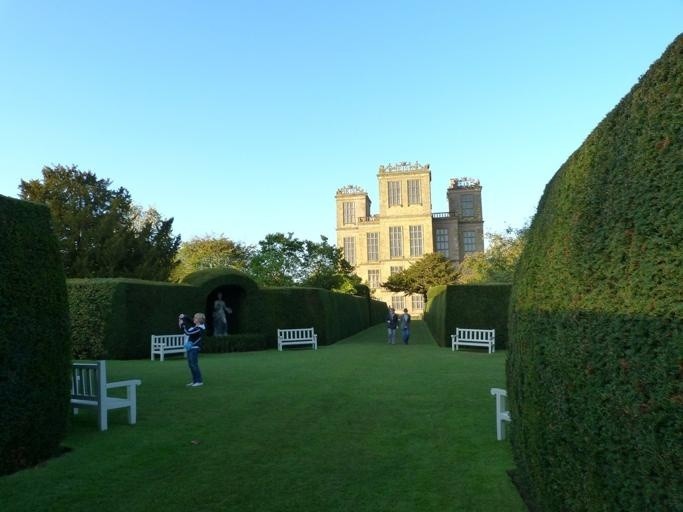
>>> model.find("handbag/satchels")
[184,341,192,351]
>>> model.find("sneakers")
[187,382,203,387]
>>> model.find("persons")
[213,293,234,336]
[179,312,208,388]
[386,307,401,344]
[400,308,412,346]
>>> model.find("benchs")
[69,360,140,435]
[274,327,318,351]
[149,332,189,362]
[449,326,495,354]
[490,386,513,440]
